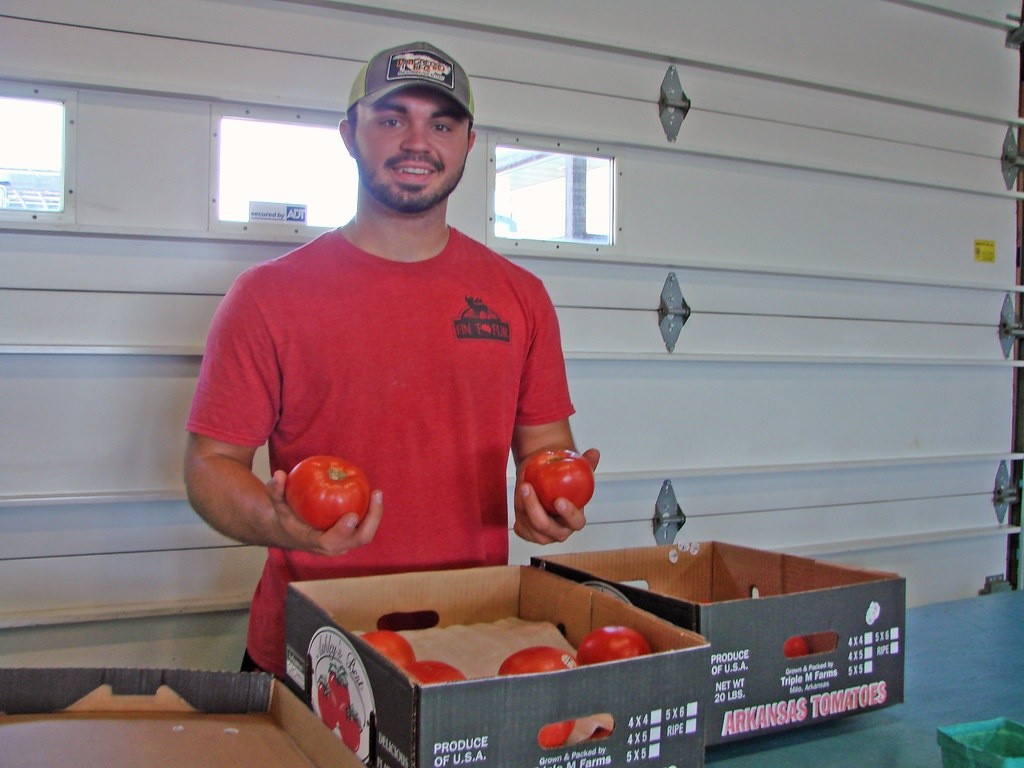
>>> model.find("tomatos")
[522,449,596,517]
[354,622,655,743]
[282,454,373,532]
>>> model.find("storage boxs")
[284,566,710,768]
[528,540,907,746]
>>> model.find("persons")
[182,40,602,682]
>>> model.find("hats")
[346,41,476,125]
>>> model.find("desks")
[704,588,1024,768]
